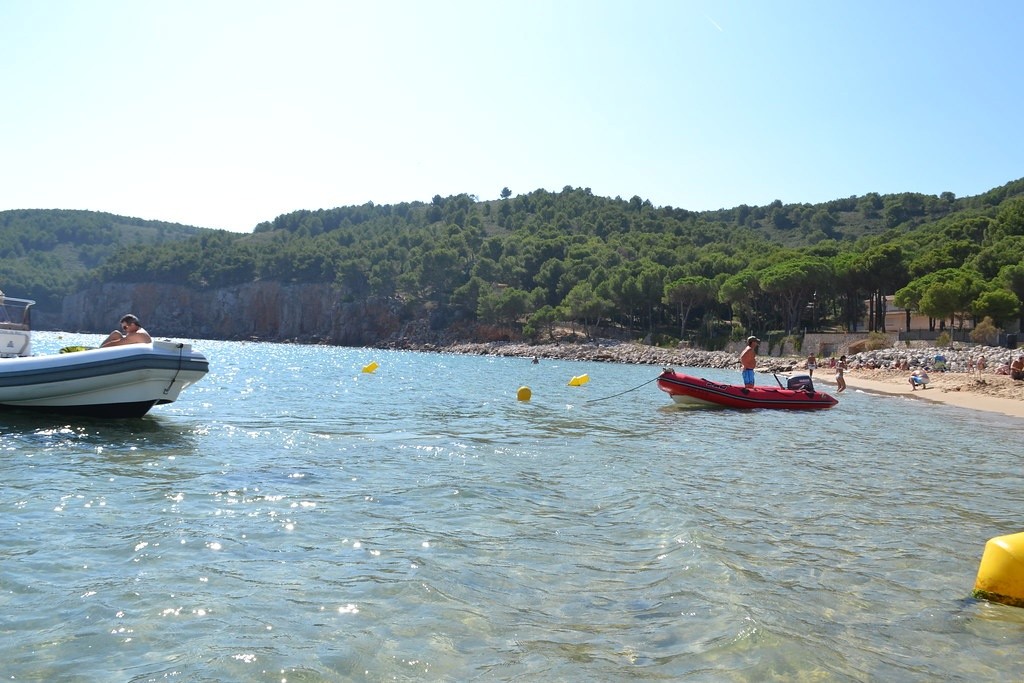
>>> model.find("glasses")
[122,322,131,331]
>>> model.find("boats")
[658,367,839,409]
[0,294,209,419]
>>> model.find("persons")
[740,336,760,388]
[941,365,946,373]
[895,360,907,372]
[967,356,974,373]
[771,363,777,373]
[1010,355,1024,379]
[99,314,151,348]
[923,364,935,373]
[908,370,929,391]
[531,356,539,364]
[856,360,874,370]
[835,355,847,392]
[817,357,836,369]
[994,362,1009,375]
[805,361,808,370]
[806,353,817,378]
[977,356,984,370]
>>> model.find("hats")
[747,336,761,344]
